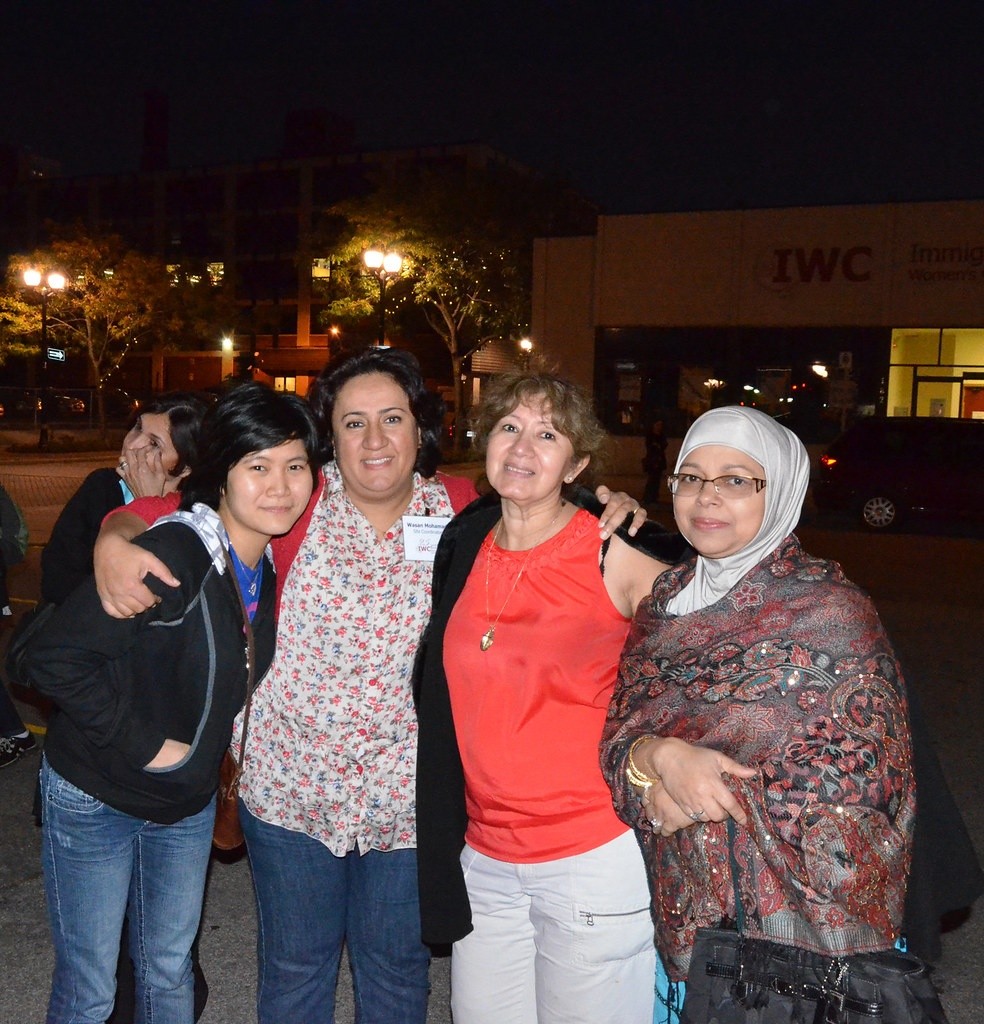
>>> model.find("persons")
[42,390,209,605]
[23,383,318,1024]
[598,405,917,1024]
[0,485,38,768]
[93,347,647,1024]
[416,352,698,1024]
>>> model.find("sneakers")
[0,728,39,768]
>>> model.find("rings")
[651,817,662,827]
[689,810,704,819]
[644,791,649,800]
[121,461,128,468]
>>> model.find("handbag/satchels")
[679,926,951,1024]
[213,749,245,851]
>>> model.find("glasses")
[666,473,766,500]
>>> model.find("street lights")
[23,269,65,450]
[364,249,402,345]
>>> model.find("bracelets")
[626,733,658,788]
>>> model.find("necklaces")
[481,496,562,650]
[226,529,259,596]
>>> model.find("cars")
[811,417,984,532]
